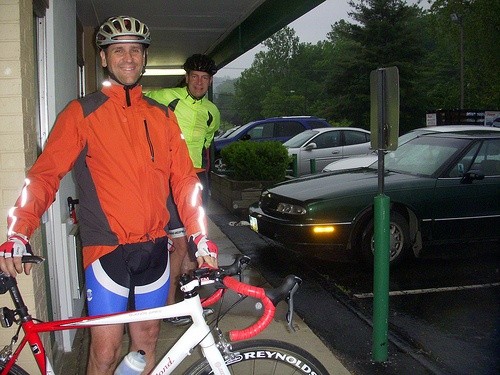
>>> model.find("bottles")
[113,349,146,375]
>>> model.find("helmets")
[184,53,217,75]
[95,15,151,46]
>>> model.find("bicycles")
[0,253,331,375]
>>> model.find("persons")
[0,15,218,375]
[142,53,221,326]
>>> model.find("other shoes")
[163,306,215,325]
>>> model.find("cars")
[321,124,500,186]
[249,130,500,267]
[279,128,376,179]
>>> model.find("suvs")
[212,117,334,161]
